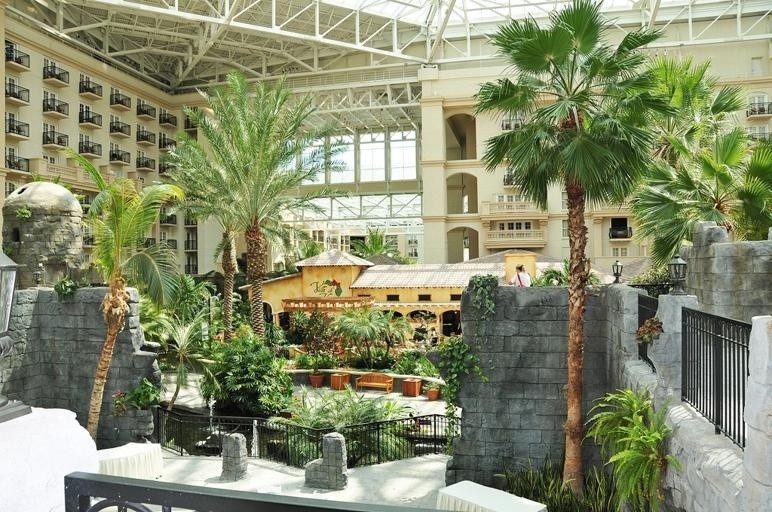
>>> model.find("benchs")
[355,372,393,394]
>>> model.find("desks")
[436,479,548,512]
[97,441,162,482]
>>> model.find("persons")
[508,263,532,287]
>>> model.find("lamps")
[611,259,624,282]
[668,254,688,295]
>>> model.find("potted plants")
[423,380,441,400]
[309,354,325,386]
